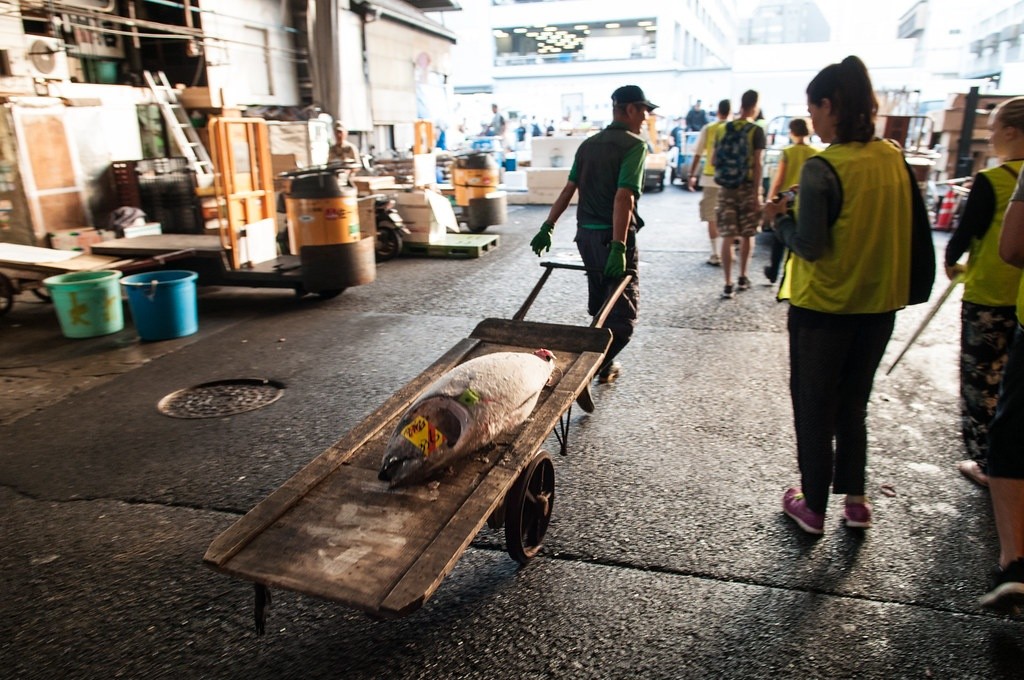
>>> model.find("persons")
[328,120,362,173]
[710,89,766,299]
[980,161,1024,614]
[530,85,660,412]
[685,99,708,132]
[688,100,737,265]
[763,119,821,284]
[764,55,936,534]
[489,104,504,136]
[944,98,1024,487]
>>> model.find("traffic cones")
[931,184,954,231]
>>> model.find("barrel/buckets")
[120,270,199,341]
[41,269,123,339]
[81,61,118,83]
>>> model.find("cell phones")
[778,190,797,207]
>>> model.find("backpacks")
[714,122,756,189]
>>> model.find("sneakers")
[980,555,1024,614]
[599,365,619,382]
[575,381,595,414]
[783,488,824,534]
[738,277,752,290]
[723,283,735,298]
[842,497,873,528]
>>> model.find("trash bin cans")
[507,159,516,172]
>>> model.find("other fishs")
[378,348,557,491]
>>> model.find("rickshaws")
[200,261,637,620]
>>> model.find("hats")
[333,120,346,133]
[611,85,659,112]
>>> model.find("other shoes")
[958,459,990,488]
[707,255,721,265]
[766,266,777,283]
[734,240,740,250]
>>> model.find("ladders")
[143,70,213,174]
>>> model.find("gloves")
[603,241,627,278]
[530,223,554,257]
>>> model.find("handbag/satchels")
[885,137,937,304]
